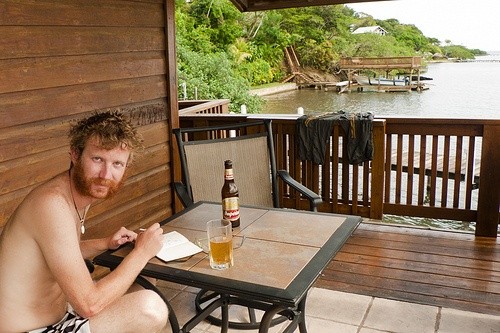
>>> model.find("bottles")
[221,160,241,228]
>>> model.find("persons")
[0,111,169,333]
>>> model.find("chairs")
[172,119,324,330]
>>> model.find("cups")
[206,219,234,270]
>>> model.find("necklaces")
[68,168,85,234]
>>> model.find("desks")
[92,199,363,333]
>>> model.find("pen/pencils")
[139,228,146,231]
